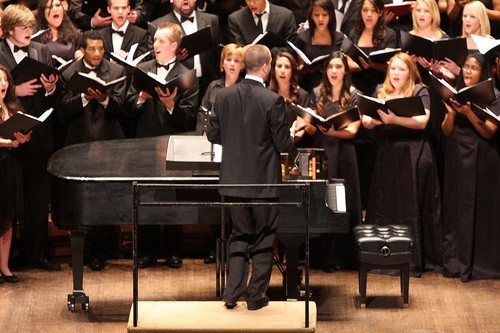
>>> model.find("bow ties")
[180,14,195,24]
[111,28,126,38]
[13,44,30,53]
[83,66,101,75]
[156,62,170,71]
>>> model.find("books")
[290,100,362,130]
[143,22,211,58]
[111,43,153,66]
[0,107,53,138]
[77,71,128,95]
[10,56,75,83]
[427,71,497,105]
[399,27,466,67]
[355,93,427,121]
[344,35,400,63]
[132,66,196,99]
[285,40,331,73]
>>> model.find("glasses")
[461,64,478,74]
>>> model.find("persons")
[207,44,296,311]
[0,0,500,284]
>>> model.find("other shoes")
[32,254,61,271]
[135,251,158,268]
[165,250,183,268]
[89,255,104,271]
[0,269,19,285]
[204,254,216,264]
[224,293,240,310]
[246,294,270,311]
[442,262,473,283]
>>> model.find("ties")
[255,12,267,35]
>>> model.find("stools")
[353,224,414,309]
